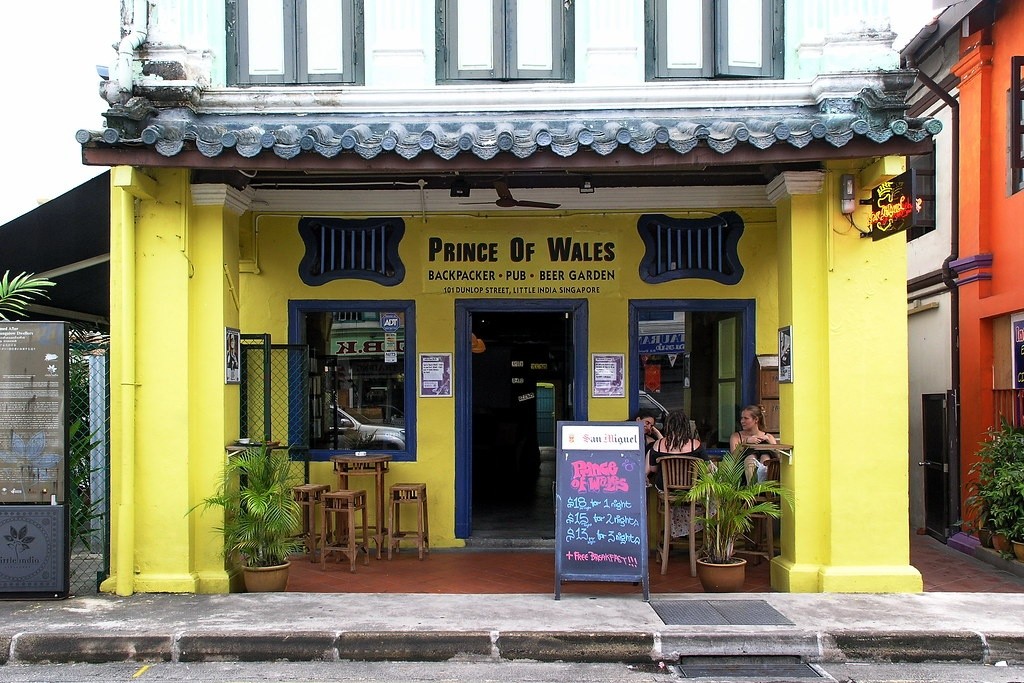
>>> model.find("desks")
[330,453,392,560]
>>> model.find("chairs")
[734,458,780,567]
[656,455,715,578]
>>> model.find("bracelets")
[746,437,748,443]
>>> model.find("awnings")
[0,168,110,334]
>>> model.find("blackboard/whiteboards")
[556,420,648,581]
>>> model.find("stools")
[320,490,368,571]
[388,482,429,561]
[292,484,333,563]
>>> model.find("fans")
[458,180,561,210]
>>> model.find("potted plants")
[950,409,1024,562]
[183,442,300,593]
[673,439,796,593]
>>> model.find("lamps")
[450,181,471,197]
[579,179,595,194]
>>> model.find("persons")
[730,405,777,486]
[227,334,239,369]
[649,409,709,506]
[626,408,664,488]
